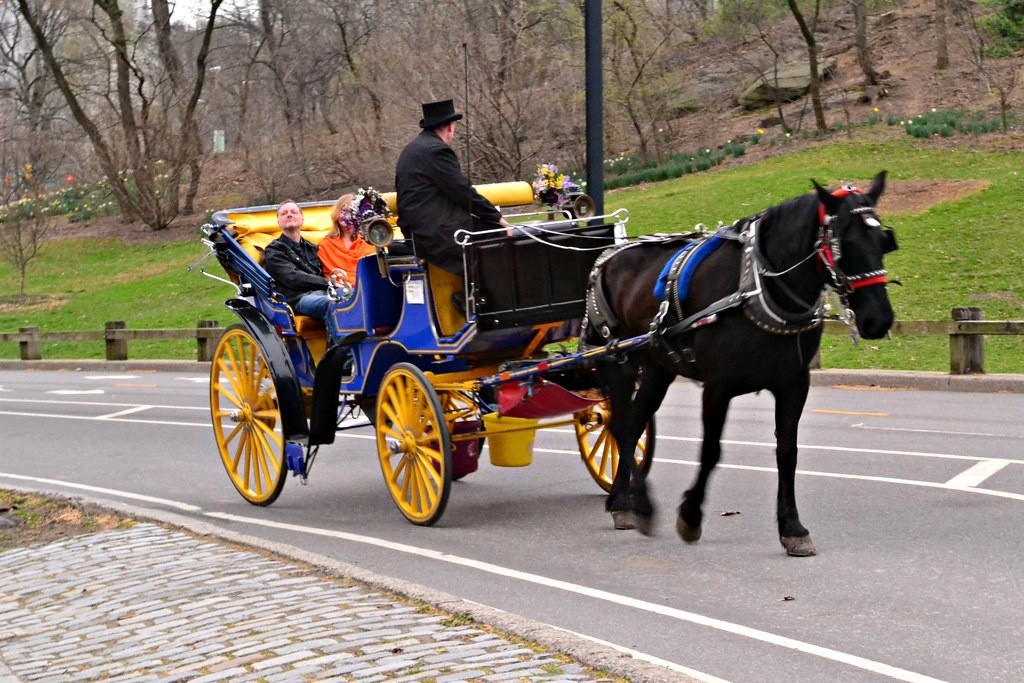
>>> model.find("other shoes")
[452,289,467,317]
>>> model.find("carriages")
[185,170,903,559]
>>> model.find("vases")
[539,187,559,207]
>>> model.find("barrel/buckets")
[423,416,480,479]
[482,409,536,467]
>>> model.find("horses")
[585,170,899,557]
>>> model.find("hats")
[418,99,463,129]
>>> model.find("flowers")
[530,161,579,211]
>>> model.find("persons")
[394,98,512,316]
[317,187,392,301]
[263,198,357,379]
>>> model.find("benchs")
[328,252,404,340]
[228,205,335,332]
